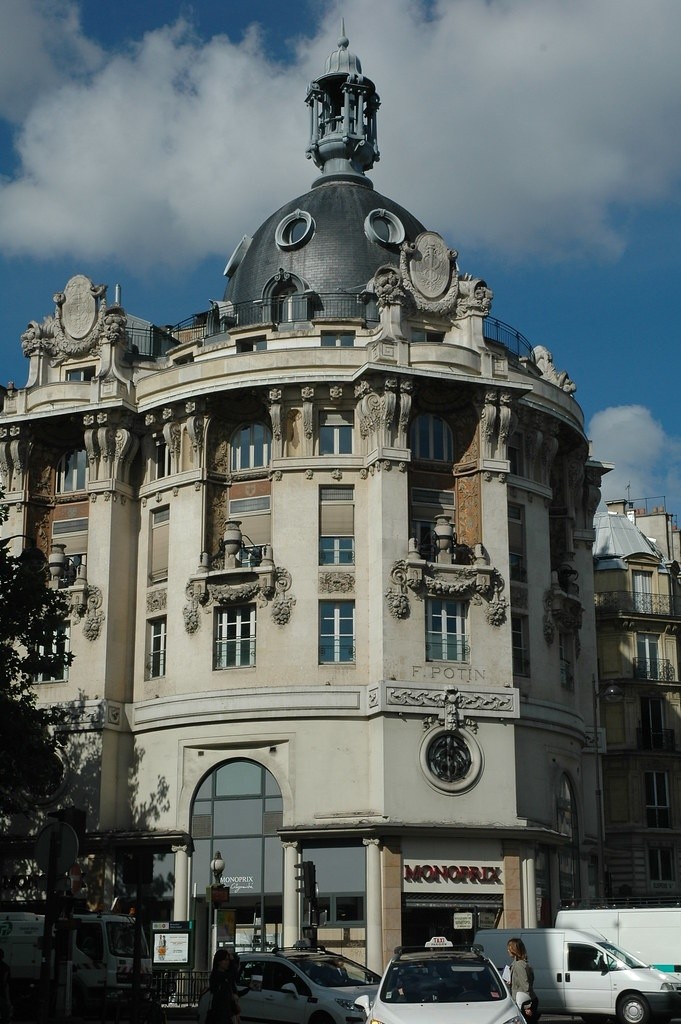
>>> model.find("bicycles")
[135,983,168,1024]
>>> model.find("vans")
[472,927,681,1024]
[554,908,681,976]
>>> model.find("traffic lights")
[294,861,316,900]
[302,925,316,939]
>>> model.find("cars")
[232,945,382,1024]
[353,937,533,1024]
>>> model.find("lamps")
[595,679,624,703]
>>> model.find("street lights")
[210,849,225,973]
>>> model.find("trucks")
[0,901,154,1020]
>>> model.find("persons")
[157,934,166,960]
[507,938,540,1024]
[210,949,262,1024]
[328,959,351,986]
[587,950,600,970]
